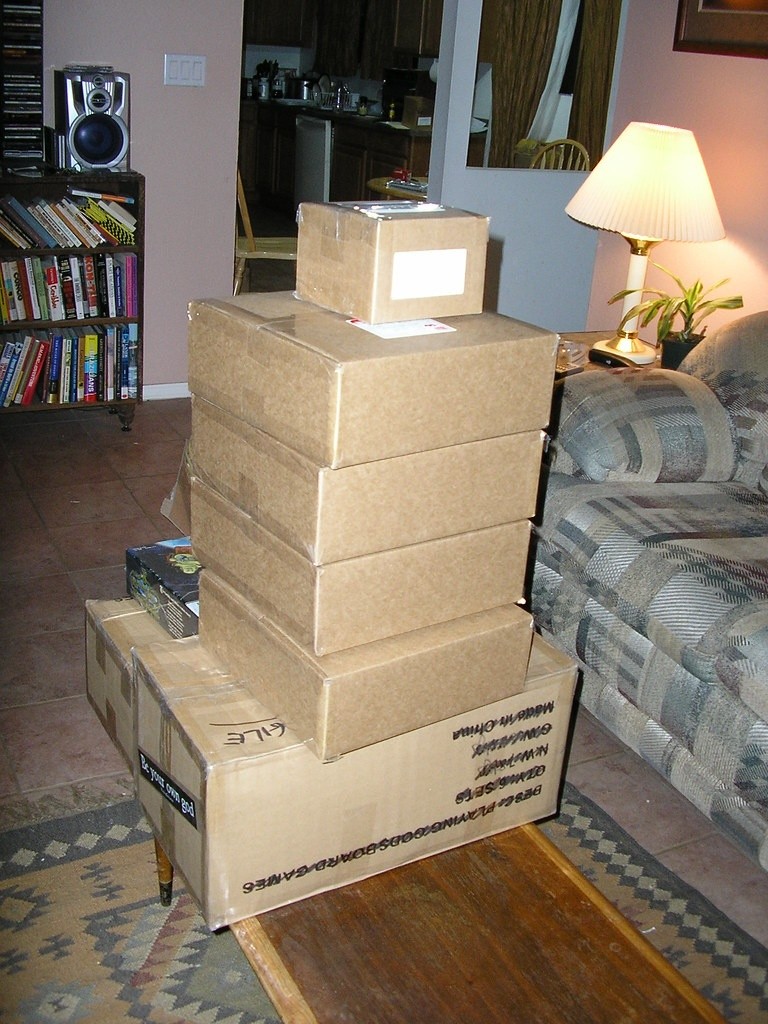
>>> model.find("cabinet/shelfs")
[0,169,147,434]
[242,0,443,77]
[256,102,295,212]
[239,99,263,207]
[329,118,495,202]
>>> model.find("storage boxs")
[81,597,177,770]
[294,196,491,328]
[133,628,579,932]
[119,533,203,641]
[197,570,534,766]
[186,292,561,470]
[402,95,436,132]
[183,478,534,659]
[187,393,548,567]
[285,78,310,100]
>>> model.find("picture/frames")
[671,0,768,62]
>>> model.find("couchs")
[524,309,768,876]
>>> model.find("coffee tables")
[155,813,729,1024]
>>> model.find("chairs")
[233,167,297,296]
[528,139,590,171]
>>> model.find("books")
[0,190,138,408]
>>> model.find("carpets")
[0,778,768,1024]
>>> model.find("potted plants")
[605,260,744,373]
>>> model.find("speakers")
[52,70,131,173]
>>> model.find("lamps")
[563,121,727,369]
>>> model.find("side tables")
[554,328,662,375]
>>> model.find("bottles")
[387,103,395,120]
[258,77,269,100]
[272,79,283,98]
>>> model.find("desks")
[367,176,429,202]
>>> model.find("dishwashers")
[293,114,334,214]
